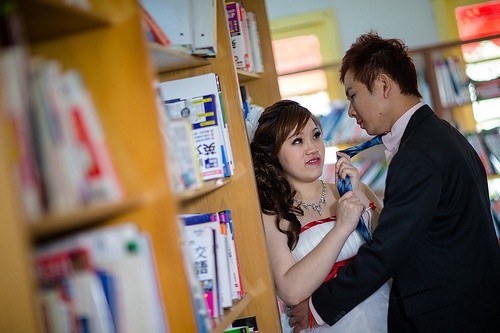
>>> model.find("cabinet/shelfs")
[278,33,500,247]
[0,0,283,333]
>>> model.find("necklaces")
[294,179,328,215]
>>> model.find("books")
[13,1,500,333]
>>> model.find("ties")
[336,132,387,243]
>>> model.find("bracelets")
[365,201,377,211]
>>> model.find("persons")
[287,30,500,333]
[250,100,390,333]
